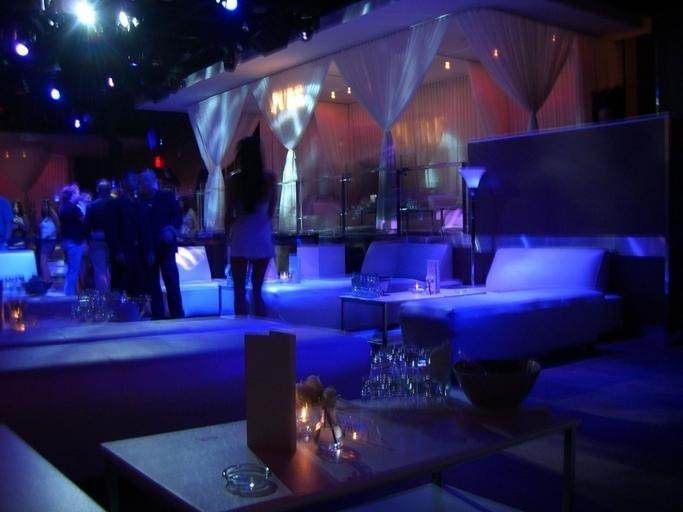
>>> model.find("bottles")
[351,272,380,297]
[425,260,436,296]
[361,343,451,402]
[121,290,129,303]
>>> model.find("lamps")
[460,165,488,287]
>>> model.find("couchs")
[400,248,623,363]
[0,318,367,414]
[246,241,460,319]
[174,247,220,315]
[2,426,100,512]
[3,249,37,281]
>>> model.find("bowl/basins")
[454,356,541,409]
[108,296,147,321]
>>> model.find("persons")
[0,170,200,319]
[223,135,277,318]
